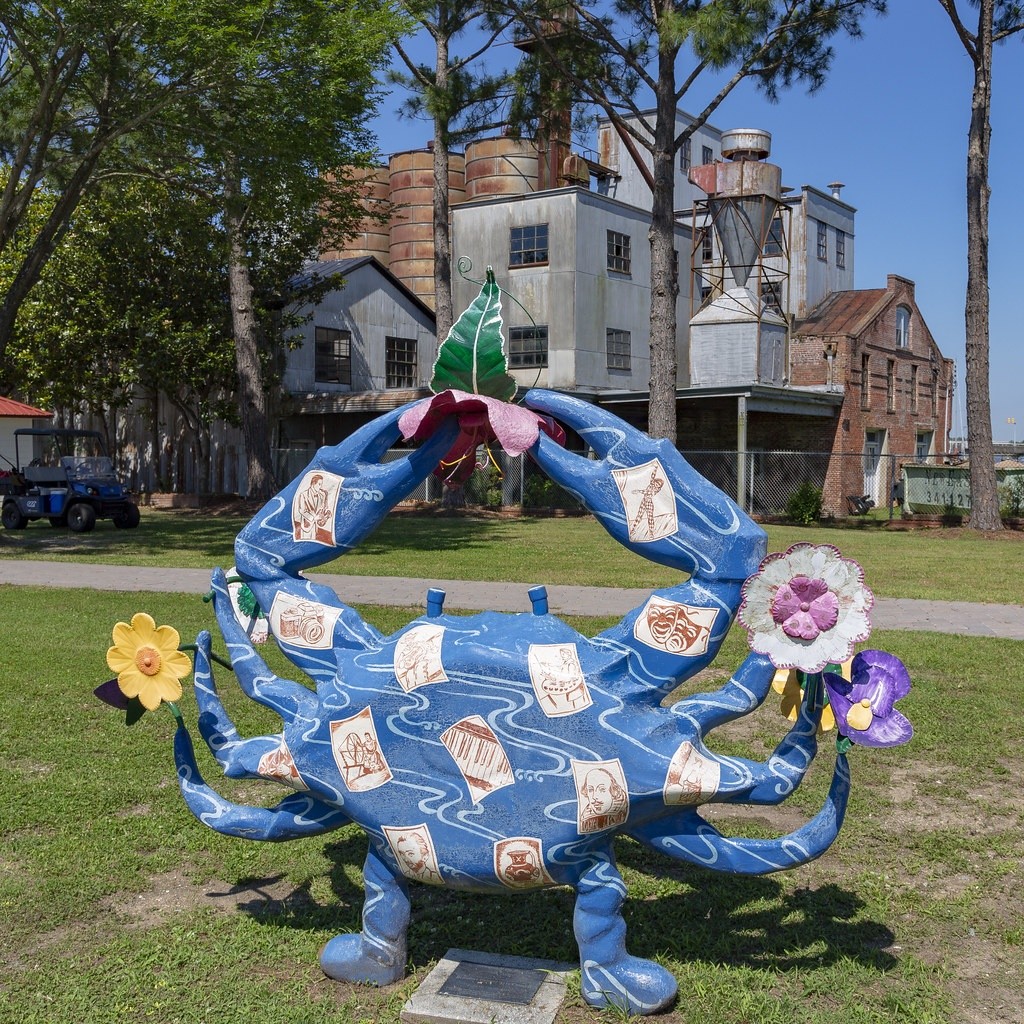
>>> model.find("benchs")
[23,465,72,487]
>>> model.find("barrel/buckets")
[49,489,66,512]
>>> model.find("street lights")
[1007,418,1017,446]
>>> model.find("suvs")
[0,426,141,532]
